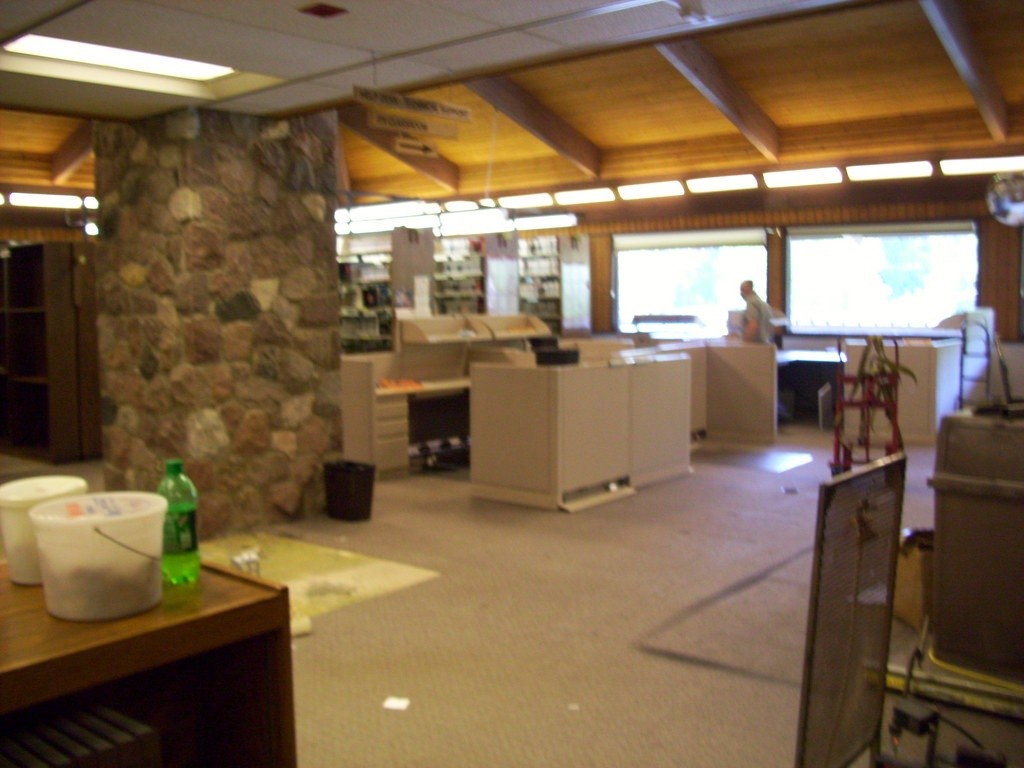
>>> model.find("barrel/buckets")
[0,475,89,585]
[28,490,167,622]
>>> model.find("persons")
[740,281,774,346]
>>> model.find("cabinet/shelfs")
[336,214,999,515]
[0,538,299,768]
[0,239,99,468]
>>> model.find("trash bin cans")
[322,460,375,522]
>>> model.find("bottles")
[156,457,202,584]
